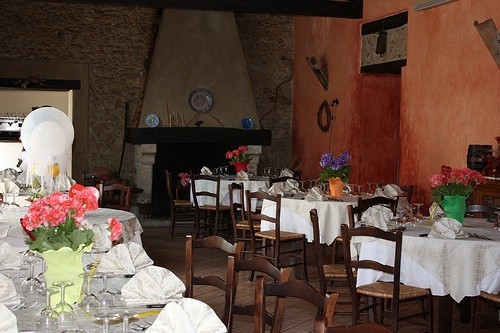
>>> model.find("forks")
[468,233,492,240]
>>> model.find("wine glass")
[366,182,380,195]
[349,184,363,193]
[307,179,330,191]
[0,192,20,211]
[393,203,424,229]
[299,181,306,192]
[21,255,139,333]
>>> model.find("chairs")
[84,168,500,333]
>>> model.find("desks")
[260,191,372,286]
[349,218,500,333]
[190,176,270,209]
[0,206,165,333]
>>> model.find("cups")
[213,166,230,177]
[263,167,276,179]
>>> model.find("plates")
[188,88,213,113]
[240,117,255,129]
[144,114,159,128]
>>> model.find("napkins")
[305,186,325,200]
[384,184,403,197]
[236,170,249,181]
[267,183,287,196]
[363,204,398,231]
[285,179,301,193]
[428,218,464,239]
[280,169,294,177]
[0,167,230,333]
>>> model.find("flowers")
[226,145,252,162]
[319,151,353,185]
[19,183,124,253]
[429,165,485,196]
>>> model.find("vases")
[30,243,94,309]
[330,177,345,197]
[466,144,493,174]
[234,162,250,171]
[442,196,466,226]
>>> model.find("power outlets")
[278,56,288,67]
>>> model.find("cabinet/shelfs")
[0,77,83,186]
[472,180,500,217]
